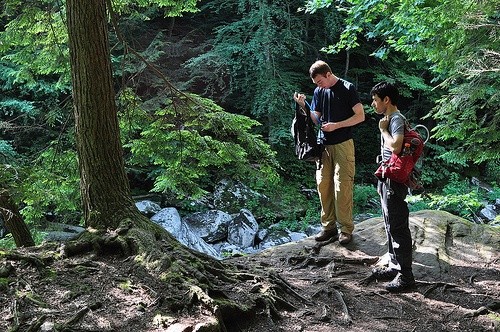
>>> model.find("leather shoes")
[314,227,338,242]
[339,231,352,244]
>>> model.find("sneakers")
[383,271,417,292]
[375,266,402,281]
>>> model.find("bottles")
[317,122,326,145]
[403,143,412,155]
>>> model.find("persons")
[294,60,365,245]
[371,82,417,293]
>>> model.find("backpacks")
[290,98,330,170]
[374,124,430,196]
